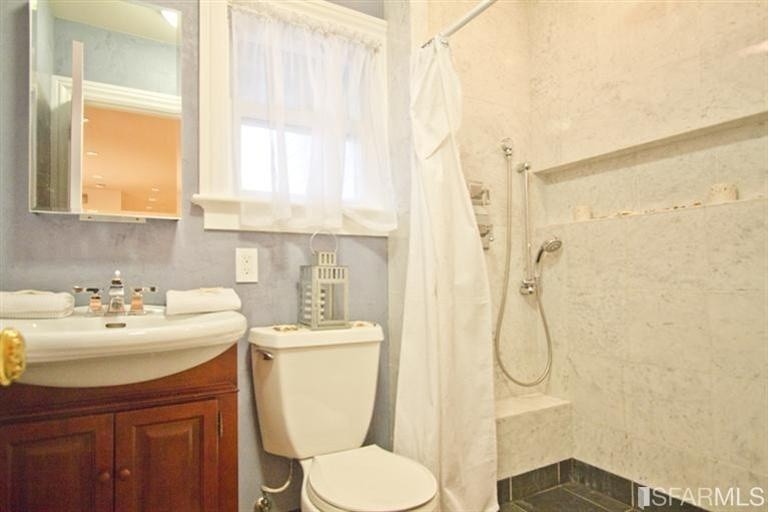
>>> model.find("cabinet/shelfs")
[0,382,238,511]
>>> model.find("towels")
[0,288,72,320]
[164,288,239,316]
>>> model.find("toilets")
[249,320,440,512]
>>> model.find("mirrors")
[28,3,186,221]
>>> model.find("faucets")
[108,286,124,314]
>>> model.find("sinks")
[0,304,247,388]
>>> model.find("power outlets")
[233,247,258,284]
